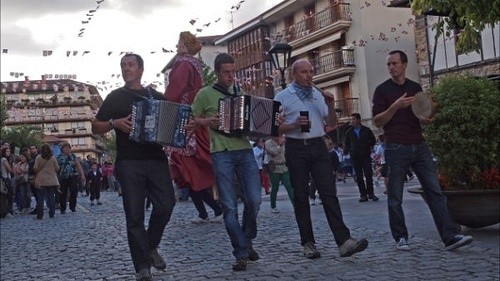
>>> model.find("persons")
[273,58,368,258]
[0,140,121,220]
[190,52,286,270]
[92,53,197,281]
[165,114,415,213]
[161,31,223,224]
[372,50,474,250]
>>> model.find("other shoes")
[135,268,152,281]
[151,250,166,269]
[303,242,320,258]
[340,238,368,256]
[359,194,378,202]
[272,208,279,213]
[248,246,258,260]
[233,257,247,271]
[311,199,322,205]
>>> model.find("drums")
[410,92,437,121]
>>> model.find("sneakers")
[191,217,209,223]
[442,234,473,250]
[209,214,224,223]
[397,238,410,250]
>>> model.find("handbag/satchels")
[264,160,276,172]
[1,177,10,195]
[28,175,36,185]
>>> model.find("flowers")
[418,69,500,188]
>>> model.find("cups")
[300,110,310,135]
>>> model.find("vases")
[407,187,500,229]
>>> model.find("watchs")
[108,119,114,130]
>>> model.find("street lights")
[266,35,293,91]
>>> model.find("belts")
[286,137,323,145]
